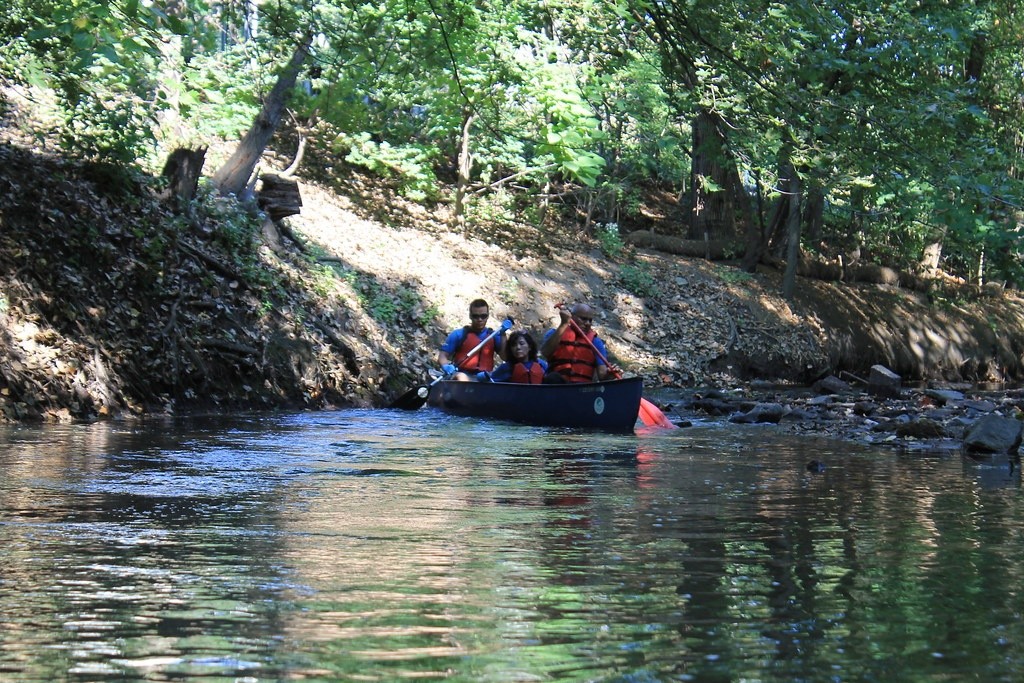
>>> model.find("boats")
[425,373,644,433]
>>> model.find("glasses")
[573,312,592,323]
[470,314,488,319]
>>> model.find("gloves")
[442,363,458,375]
[500,319,512,333]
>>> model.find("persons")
[477,330,548,384]
[539,300,625,384]
[438,298,512,382]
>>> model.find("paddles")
[552,300,673,426]
[387,314,515,410]
